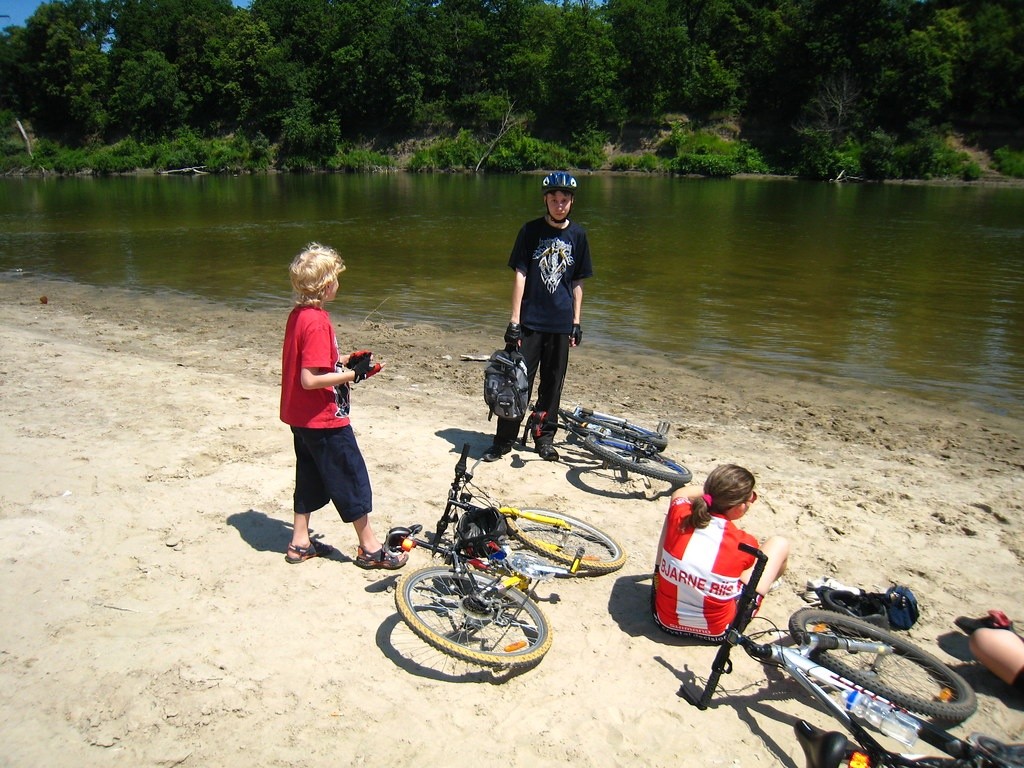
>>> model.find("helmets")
[542,171,578,195]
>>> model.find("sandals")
[285,536,334,564]
[356,543,409,569]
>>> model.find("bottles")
[839,689,922,747]
[582,422,611,436]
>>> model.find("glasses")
[728,491,758,511]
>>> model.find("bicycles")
[384,442,627,672]
[520,402,693,484]
[679,542,1024,768]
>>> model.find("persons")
[969,627,1024,698]
[279,241,410,570]
[651,464,789,644]
[481,172,593,462]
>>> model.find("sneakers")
[535,443,559,460]
[803,575,860,603]
[482,445,512,462]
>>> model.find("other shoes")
[766,577,783,591]
[954,610,1014,635]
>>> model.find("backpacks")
[885,580,919,630]
[483,344,529,422]
